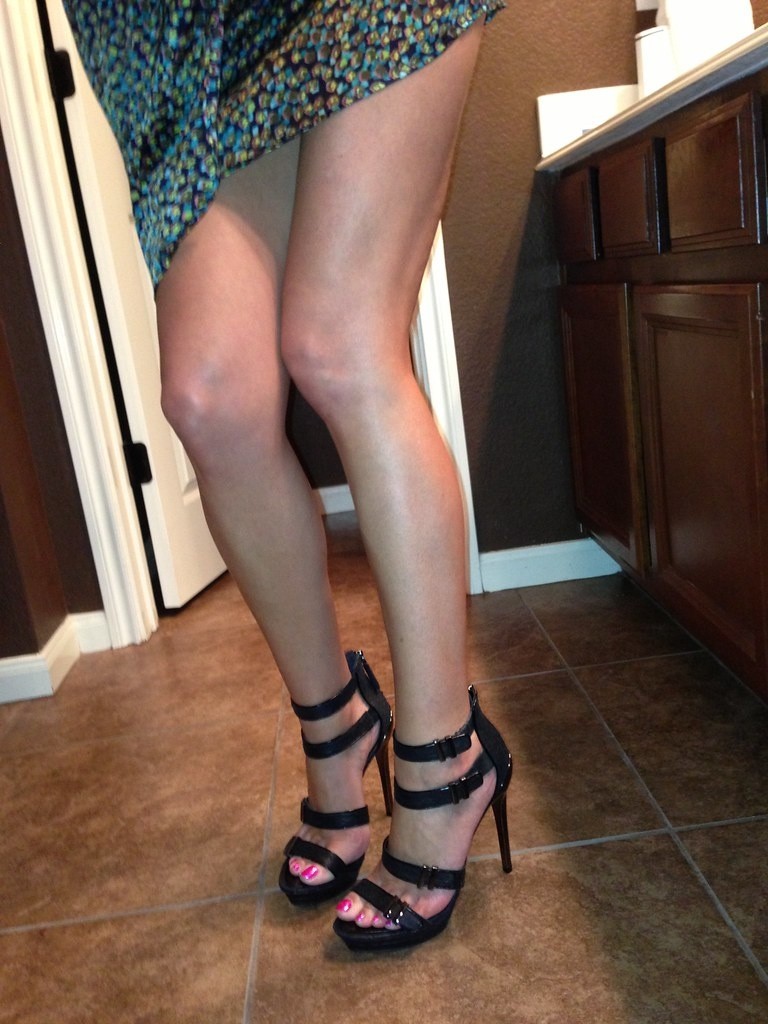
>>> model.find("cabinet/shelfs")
[549,61,768,703]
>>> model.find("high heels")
[278,652,395,908]
[332,685,515,952]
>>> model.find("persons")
[64,0,515,953]
